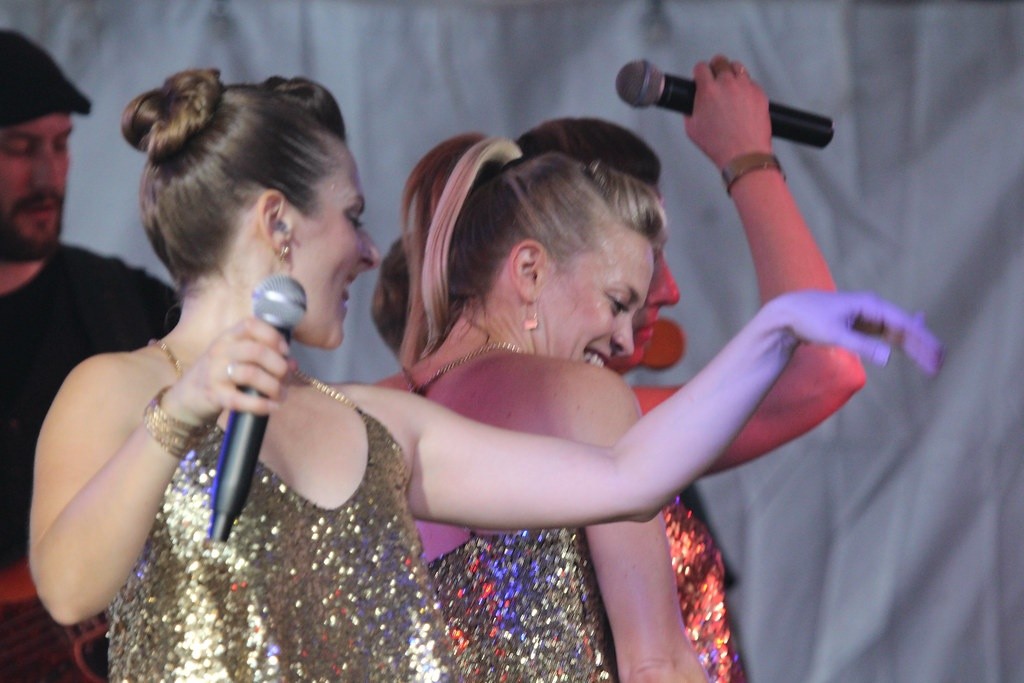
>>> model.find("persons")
[29,64,937,683]
[0,27,181,683]
[370,131,741,683]
[371,52,865,590]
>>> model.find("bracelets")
[722,152,786,195]
[144,387,208,460]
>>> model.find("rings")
[711,60,732,79]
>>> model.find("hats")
[0,29,90,127]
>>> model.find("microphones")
[617,61,835,149]
[210,275,306,544]
